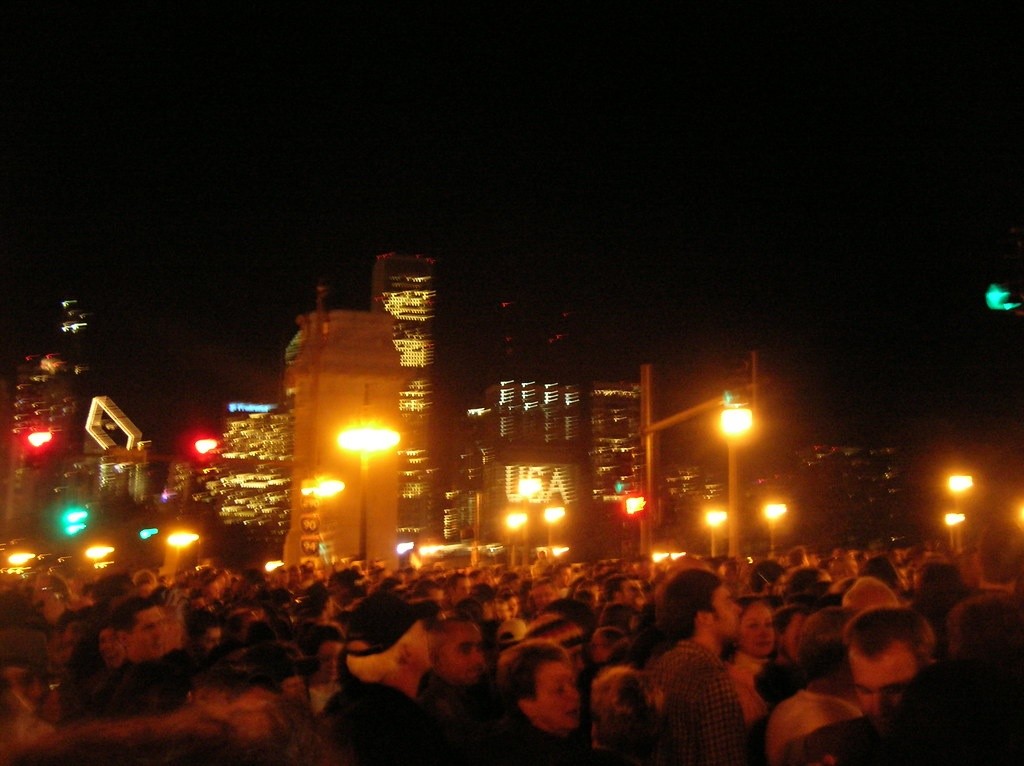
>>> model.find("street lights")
[948,474,973,553]
[346,427,392,560]
[763,504,789,552]
[517,479,541,567]
[945,514,958,549]
[706,511,726,558]
[718,408,754,558]
[543,506,562,548]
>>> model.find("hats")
[496,620,532,645]
[785,565,835,598]
[346,588,434,655]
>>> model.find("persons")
[0,538,1024,766]
[319,588,459,766]
